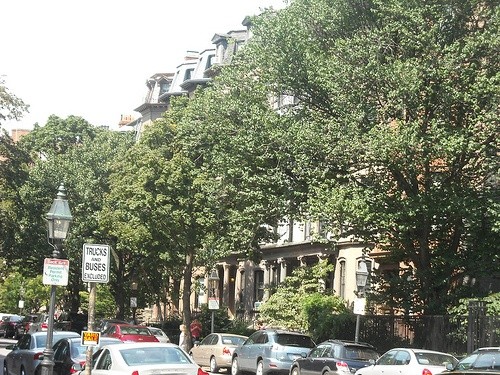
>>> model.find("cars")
[189,329,500,375]
[0,310,209,375]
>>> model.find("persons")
[190,316,202,347]
[179,325,191,354]
[258,321,266,343]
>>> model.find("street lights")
[18,286,25,317]
[131,278,139,320]
[208,266,220,332]
[42,182,72,374]
[352,254,368,342]
